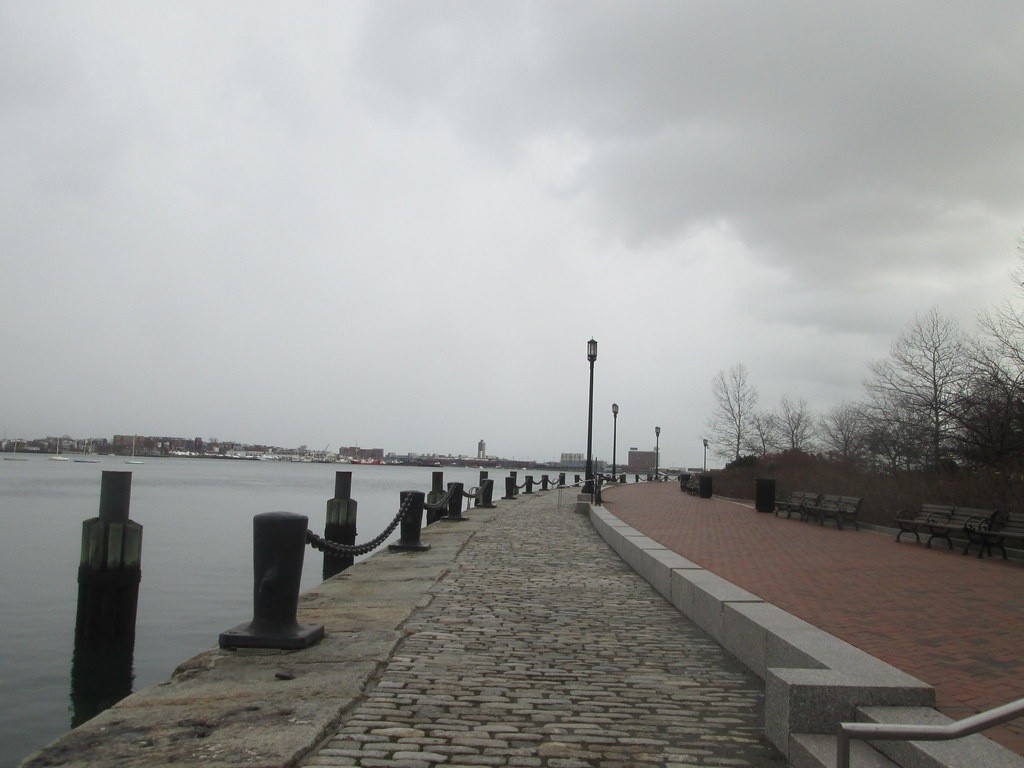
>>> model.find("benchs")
[829,496,862,531]
[893,503,955,548]
[774,493,804,519]
[975,512,1024,560]
[923,506,996,556]
[787,493,822,522]
[804,494,843,527]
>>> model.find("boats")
[74,460,102,463]
[3,458,28,461]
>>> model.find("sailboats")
[125,438,144,464]
[48,437,69,461]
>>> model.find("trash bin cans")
[698,475,713,499]
[755,477,777,514]
[680,472,691,491]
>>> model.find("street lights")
[612,404,618,481]
[585,339,597,483]
[703,439,708,471]
[655,427,660,480]
